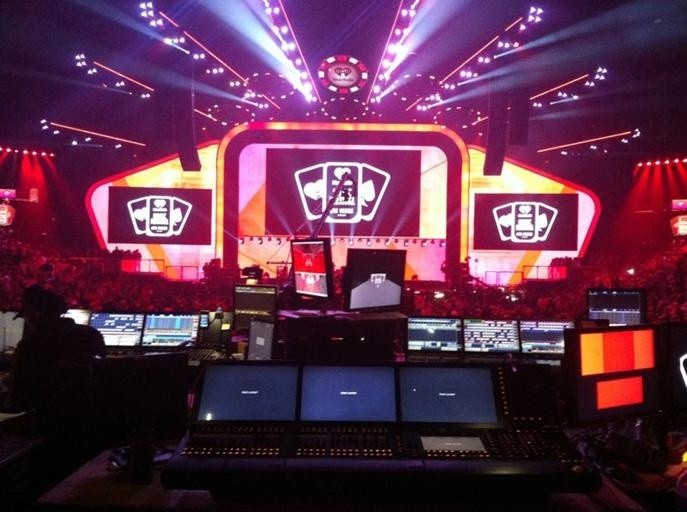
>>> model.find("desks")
[38,442,647,512]
[0,433,46,512]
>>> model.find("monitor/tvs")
[584,288,646,327]
[246,318,277,360]
[565,325,667,428]
[60,307,93,326]
[462,317,521,355]
[297,360,400,428]
[575,319,609,329]
[139,312,201,349]
[397,362,504,429]
[405,316,463,353]
[344,249,407,319]
[233,285,278,342]
[87,311,146,349]
[190,360,301,428]
[291,238,332,318]
[518,319,577,355]
[667,322,687,410]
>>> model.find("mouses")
[616,462,632,480]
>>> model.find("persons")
[0,233,237,316]
[1,285,72,414]
[405,232,687,324]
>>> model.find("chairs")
[18,326,105,432]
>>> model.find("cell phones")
[199,312,209,329]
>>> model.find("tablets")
[418,433,493,460]
